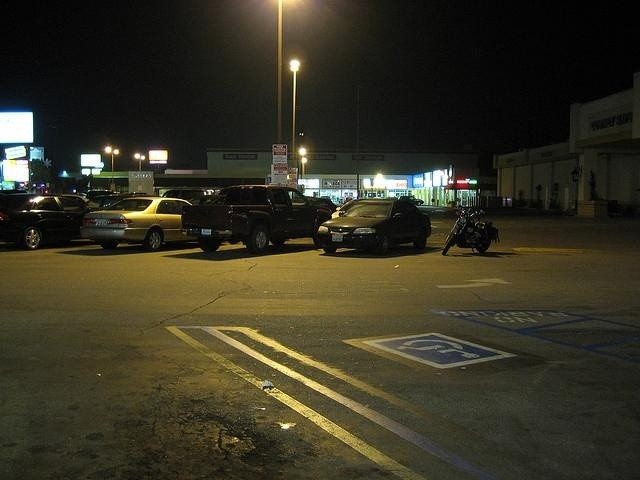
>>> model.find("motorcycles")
[440,198,501,257]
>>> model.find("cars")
[301,193,357,219]
[315,198,431,254]
[81,196,198,250]
[59,186,216,210]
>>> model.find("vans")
[0,192,96,250]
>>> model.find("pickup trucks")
[180,182,336,253]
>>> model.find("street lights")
[106,144,120,189]
[289,56,302,172]
[134,152,146,171]
[296,127,307,179]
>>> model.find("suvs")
[399,196,425,206]
